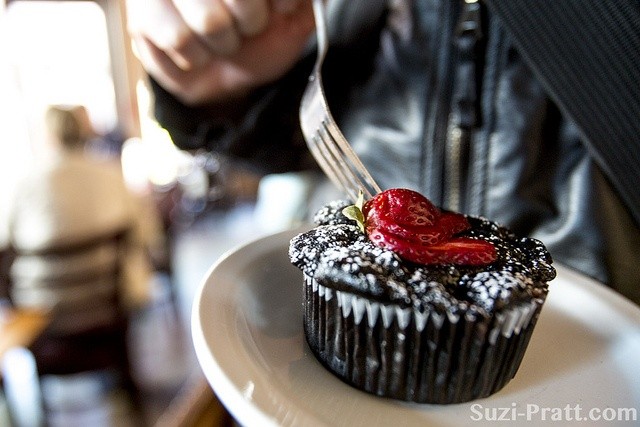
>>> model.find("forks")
[297,0,384,203]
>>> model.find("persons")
[4,104,163,319]
[123,0,640,309]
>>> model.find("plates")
[192,222,639,427]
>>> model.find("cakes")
[289,189,558,406]
